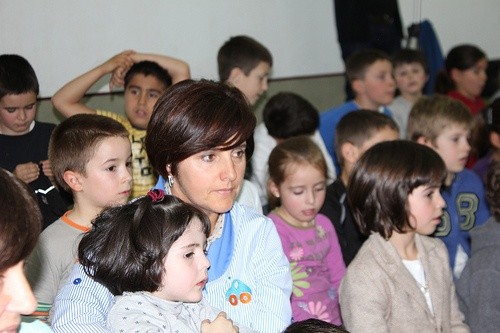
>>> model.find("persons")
[52,77,292,333]
[216,35,273,181]
[317,49,397,178]
[78,189,239,333]
[1,54,74,232]
[23,113,133,326]
[318,109,400,268]
[339,140,470,333]
[456,96,500,333]
[52,50,191,202]
[267,137,347,333]
[406,96,491,281]
[0,168,38,333]
[445,44,488,116]
[283,318,351,333]
[249,91,338,215]
[388,52,431,139]
